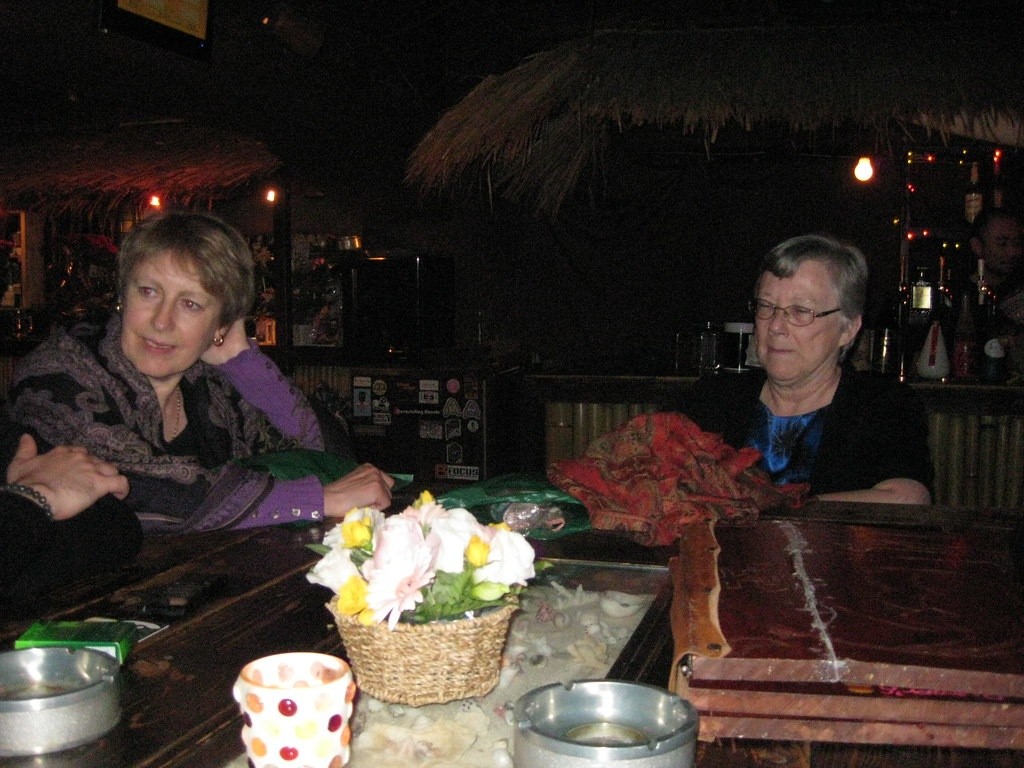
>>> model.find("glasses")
[746,298,842,326]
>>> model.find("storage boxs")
[14,619,138,666]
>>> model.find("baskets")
[326,598,514,709]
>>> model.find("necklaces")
[171,385,181,441]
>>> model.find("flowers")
[305,488,556,632]
[81,233,119,267]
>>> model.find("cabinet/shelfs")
[348,363,524,481]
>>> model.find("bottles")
[951,295,978,383]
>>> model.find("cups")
[232,652,357,768]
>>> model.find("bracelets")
[0,484,52,518]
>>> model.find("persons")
[952,207,1024,347]
[0,415,144,616]
[655,236,931,507]
[5,210,395,529]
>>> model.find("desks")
[0,481,1024,768]
